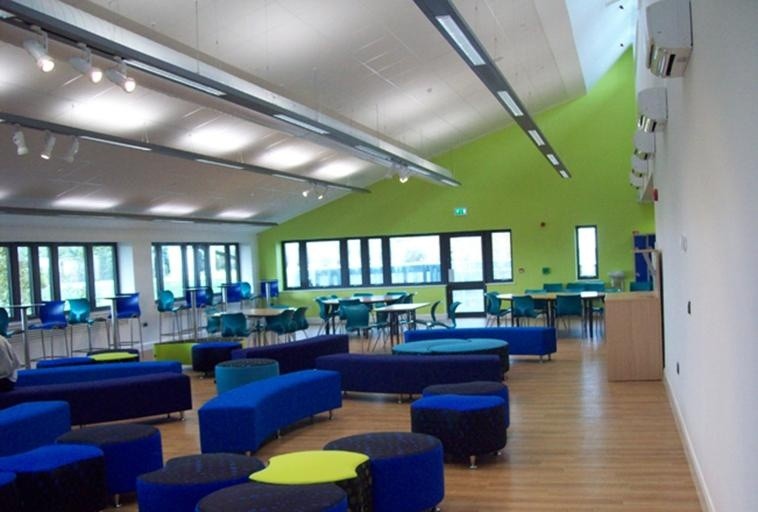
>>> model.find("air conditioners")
[626,0,693,204]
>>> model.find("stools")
[0,324,556,511]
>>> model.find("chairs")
[0,278,619,365]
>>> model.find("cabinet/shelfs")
[604,251,664,383]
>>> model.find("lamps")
[5,125,83,166]
[386,157,416,185]
[297,182,337,201]
[20,24,141,94]
